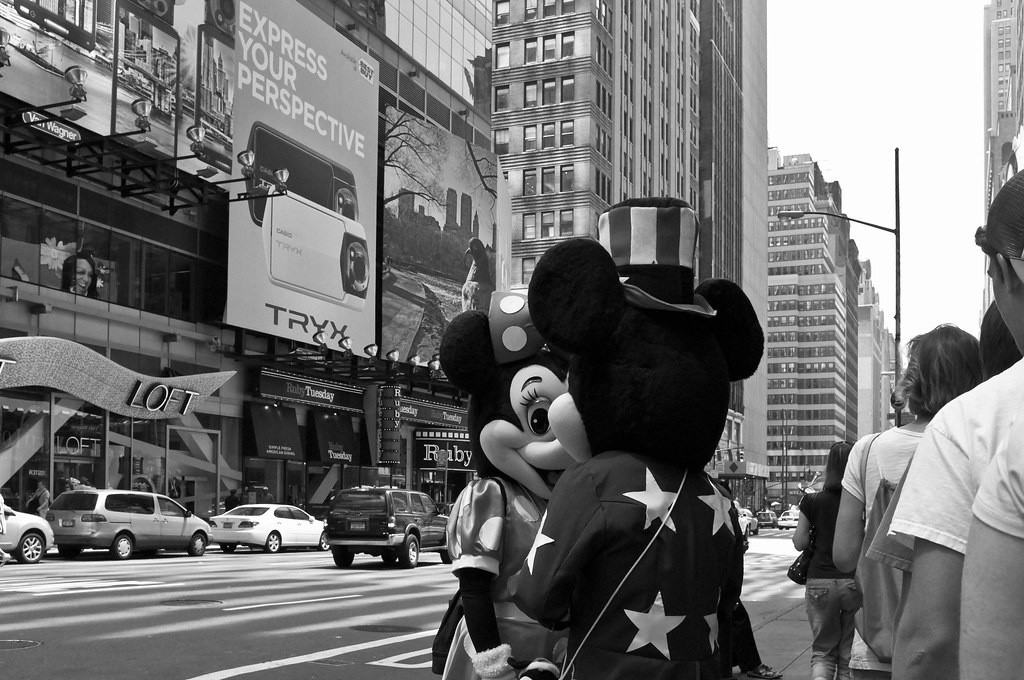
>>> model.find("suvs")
[327,485,454,562]
[756,511,778,529]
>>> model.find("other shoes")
[747,662,782,679]
[0,553,11,567]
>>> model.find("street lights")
[777,148,903,429]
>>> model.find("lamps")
[236,331,451,384]
[1,29,290,211]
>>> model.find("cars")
[45,489,214,561]
[0,504,55,564]
[777,510,801,530]
[208,503,331,553]
[738,508,759,540]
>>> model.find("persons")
[259,486,274,503]
[60,253,98,298]
[225,489,243,512]
[792,440,862,680]
[731,598,782,680]
[0,491,11,567]
[833,168,1024,680]
[162,469,182,498]
[26,479,50,519]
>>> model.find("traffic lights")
[738,449,744,462]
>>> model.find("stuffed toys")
[438,196,764,680]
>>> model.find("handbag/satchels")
[788,491,819,585]
[29,489,46,512]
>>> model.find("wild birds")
[460,237,495,316]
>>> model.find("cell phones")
[243,121,370,312]
